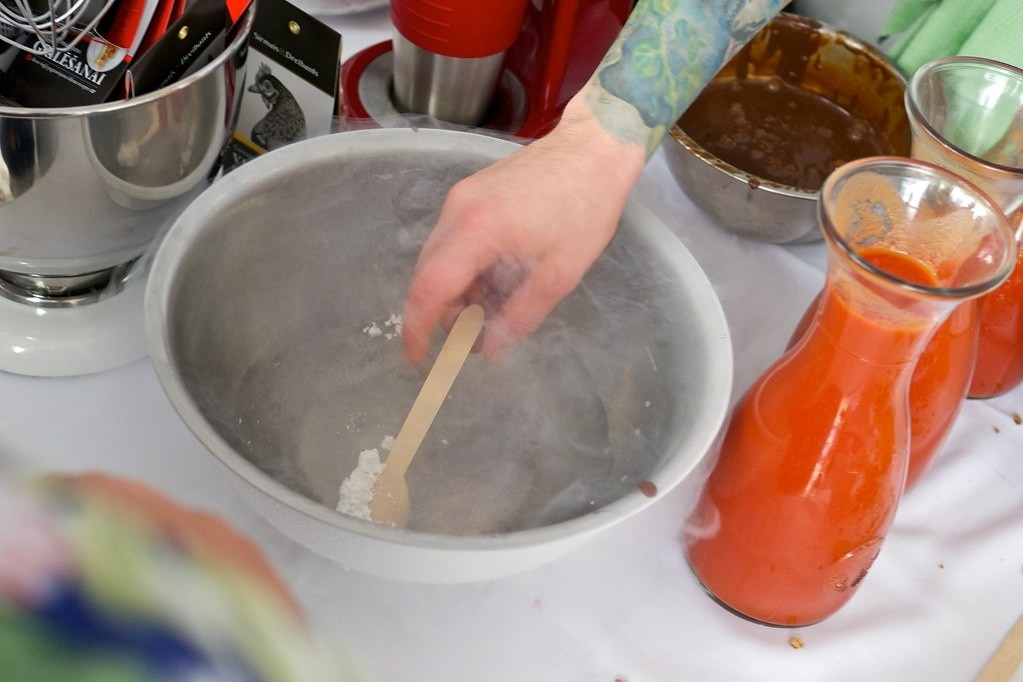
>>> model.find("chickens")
[245,63,306,153]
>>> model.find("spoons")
[373,304,485,530]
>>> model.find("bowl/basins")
[662,12,912,245]
[145,127,733,582]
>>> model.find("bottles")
[682,156,1017,627]
[967,238,1023,399]
[785,56,1023,492]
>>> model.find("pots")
[0,0,256,272]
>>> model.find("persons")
[403,0,797,365]
